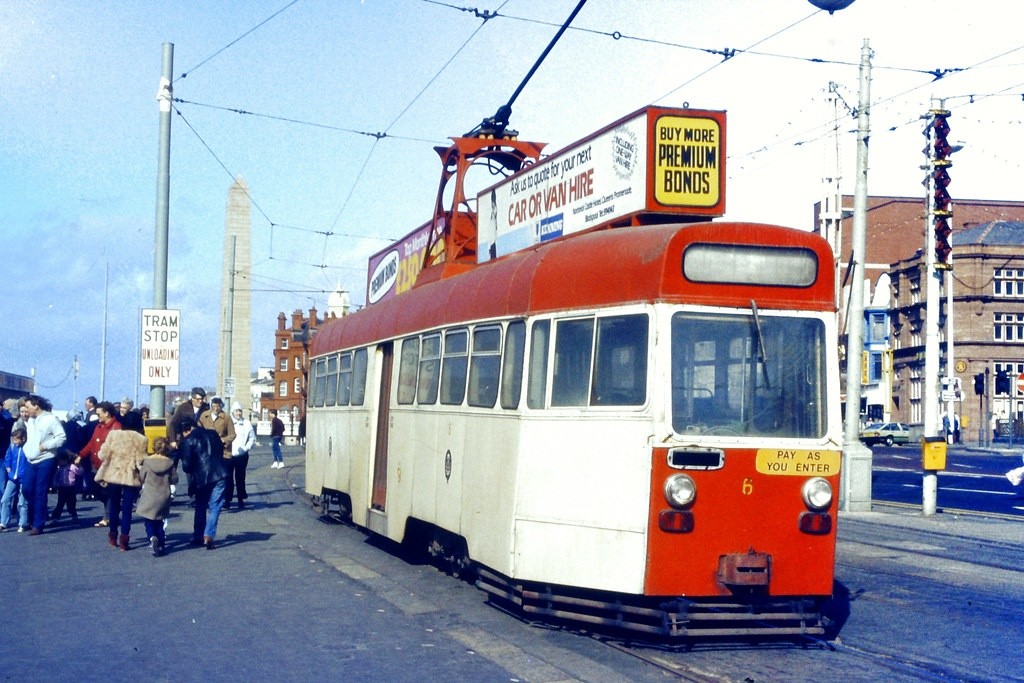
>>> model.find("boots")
[119,534,131,551]
[107,532,119,547]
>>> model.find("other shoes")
[223,502,231,509]
[238,502,245,509]
[0,524,7,532]
[189,537,203,547]
[17,525,25,532]
[203,536,216,550]
[71,517,80,524]
[94,519,110,527]
[150,536,159,558]
[26,527,43,536]
[46,520,59,528]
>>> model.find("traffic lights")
[974,373,985,396]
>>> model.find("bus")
[291,0,853,651]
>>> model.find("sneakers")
[277,461,285,469]
[270,461,279,469]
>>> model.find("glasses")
[193,397,204,401]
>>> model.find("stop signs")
[1016,373,1024,394]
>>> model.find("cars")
[858,422,910,448]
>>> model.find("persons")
[943,412,959,443]
[268,409,284,469]
[0,395,66,535]
[170,387,255,549]
[50,396,178,556]
